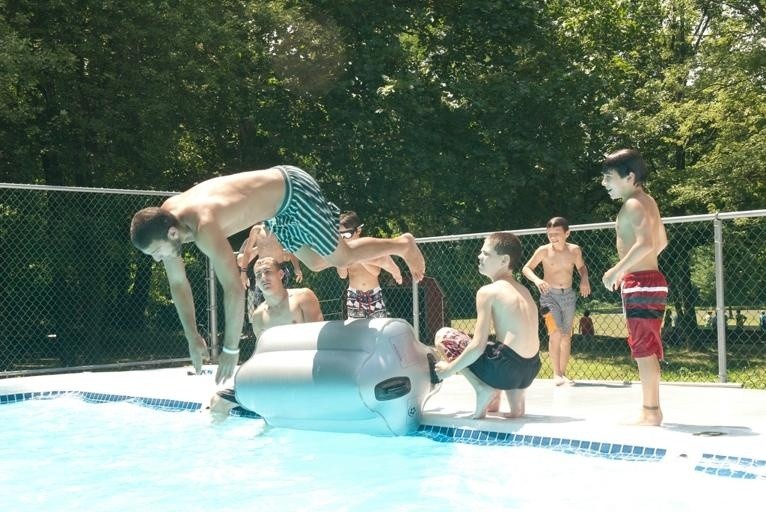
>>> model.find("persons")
[735,310,748,326]
[759,310,766,327]
[724,311,729,327]
[702,311,713,328]
[599,149,671,427]
[130,163,427,389]
[432,231,542,420]
[251,255,324,339]
[711,311,717,327]
[230,222,303,289]
[335,207,387,320]
[579,310,594,336]
[522,214,591,386]
[664,309,672,327]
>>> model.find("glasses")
[339,229,356,239]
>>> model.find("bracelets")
[221,347,241,356]
[240,267,249,273]
